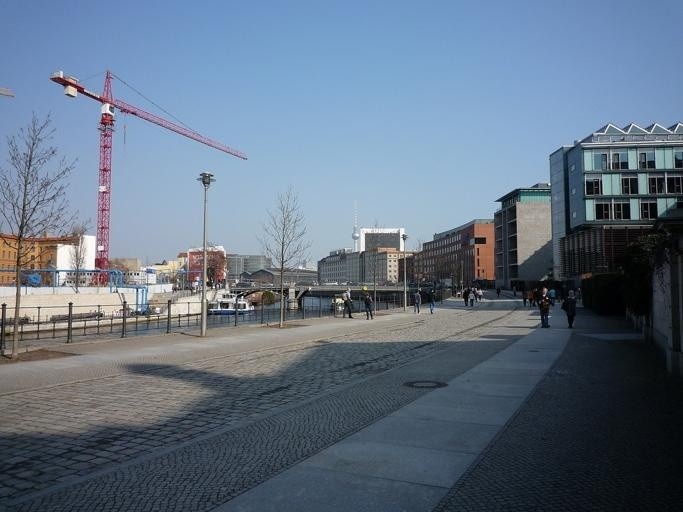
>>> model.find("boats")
[207,293,255,315]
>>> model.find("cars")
[236,279,433,288]
[138,306,151,315]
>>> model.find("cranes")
[50,66,248,287]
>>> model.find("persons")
[513,286,516,296]
[462,285,482,306]
[565,290,576,328]
[541,296,554,328]
[496,287,501,297]
[414,290,421,313]
[364,293,373,319]
[342,287,352,318]
[538,286,548,321]
[429,296,435,314]
[520,285,556,306]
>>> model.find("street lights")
[197,172,216,337]
[401,234,408,311]
